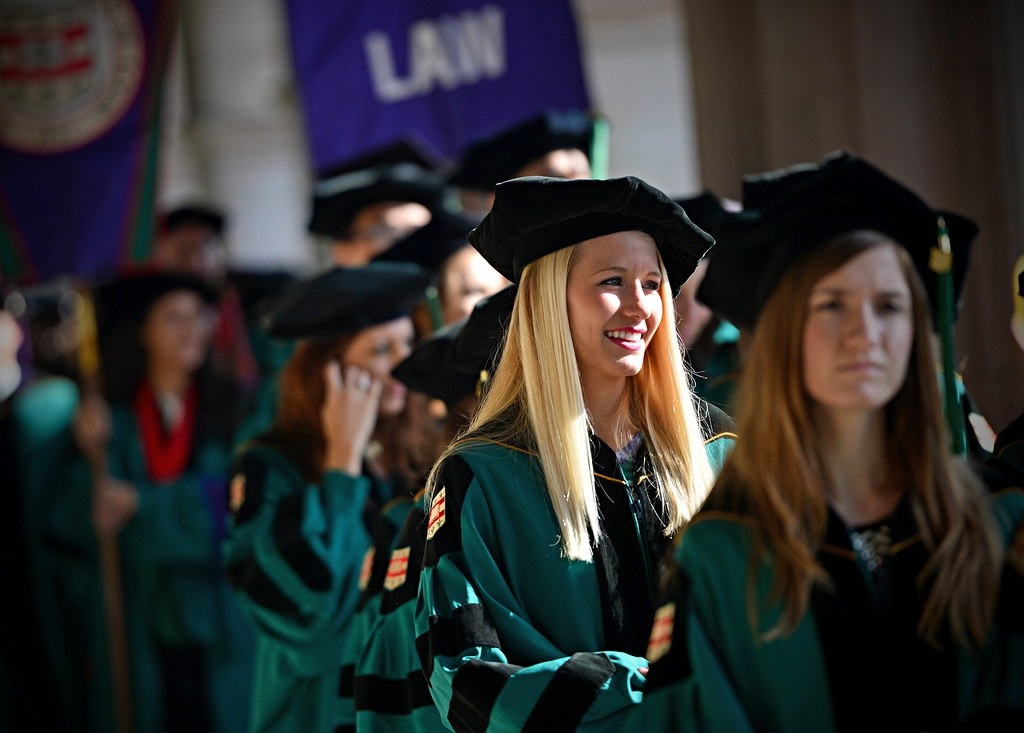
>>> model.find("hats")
[262,257,427,339]
[301,105,595,268]
[97,200,298,323]
[390,284,525,401]
[465,177,717,301]
[738,152,980,457]
[672,192,745,313]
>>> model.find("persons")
[0,113,1024,733]
